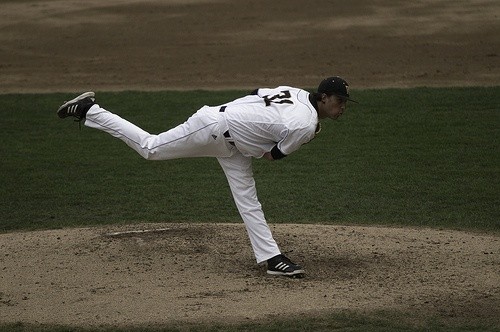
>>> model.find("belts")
[217,103,236,148]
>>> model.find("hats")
[318,76,360,103]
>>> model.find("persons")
[56,76,359,276]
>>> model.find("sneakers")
[266,254,305,276]
[57,91,96,129]
